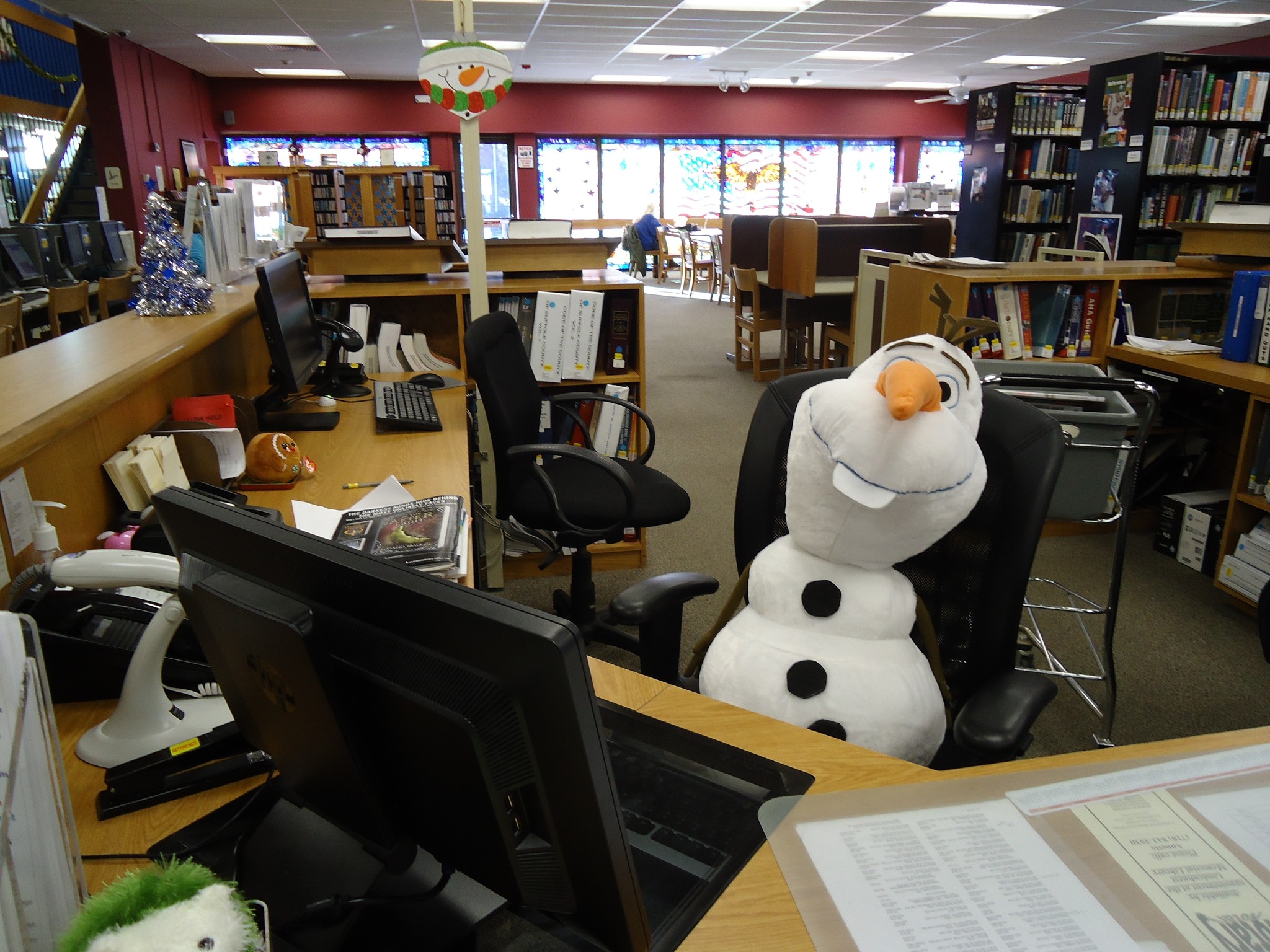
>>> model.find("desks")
[0,270,143,347]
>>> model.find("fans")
[913,75,974,108]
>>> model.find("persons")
[970,92,997,203]
[1082,73,1134,263]
[632,201,680,279]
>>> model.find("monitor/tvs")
[54,222,88,283]
[251,251,340,433]
[98,221,129,278]
[0,234,44,291]
[148,486,655,952]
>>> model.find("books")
[507,219,574,240]
[999,81,1088,265]
[310,300,460,374]
[169,186,234,238]
[898,182,962,257]
[497,290,641,560]
[329,495,473,585]
[1139,57,1269,273]
[962,271,1270,611]
[311,170,458,247]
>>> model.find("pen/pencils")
[342,480,415,489]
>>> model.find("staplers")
[95,719,276,823]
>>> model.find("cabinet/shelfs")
[878,260,1234,537]
[1103,338,1270,614]
[307,277,648,574]
[1067,52,1270,259]
[212,164,457,242]
[956,80,1091,257]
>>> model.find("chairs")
[90,272,133,327]
[32,280,91,346]
[0,295,27,353]
[607,361,1063,769]
[463,309,691,667]
[624,214,911,385]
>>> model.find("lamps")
[713,69,753,93]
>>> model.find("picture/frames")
[1036,243,1104,261]
[180,138,201,178]
[171,167,183,191]
[517,145,534,169]
[1072,212,1124,261]
[258,151,279,167]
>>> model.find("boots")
[653,264,668,278]
[669,259,680,267]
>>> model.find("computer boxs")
[32,224,87,280]
[0,226,57,288]
[86,221,110,271]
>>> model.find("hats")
[415,40,513,74]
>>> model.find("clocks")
[104,166,123,190]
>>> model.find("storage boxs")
[968,349,1141,522]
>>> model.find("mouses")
[408,373,444,389]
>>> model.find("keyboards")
[595,703,789,882]
[19,292,46,304]
[373,381,443,434]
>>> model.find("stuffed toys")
[58,851,268,951]
[246,431,303,483]
[681,279,1001,770]
